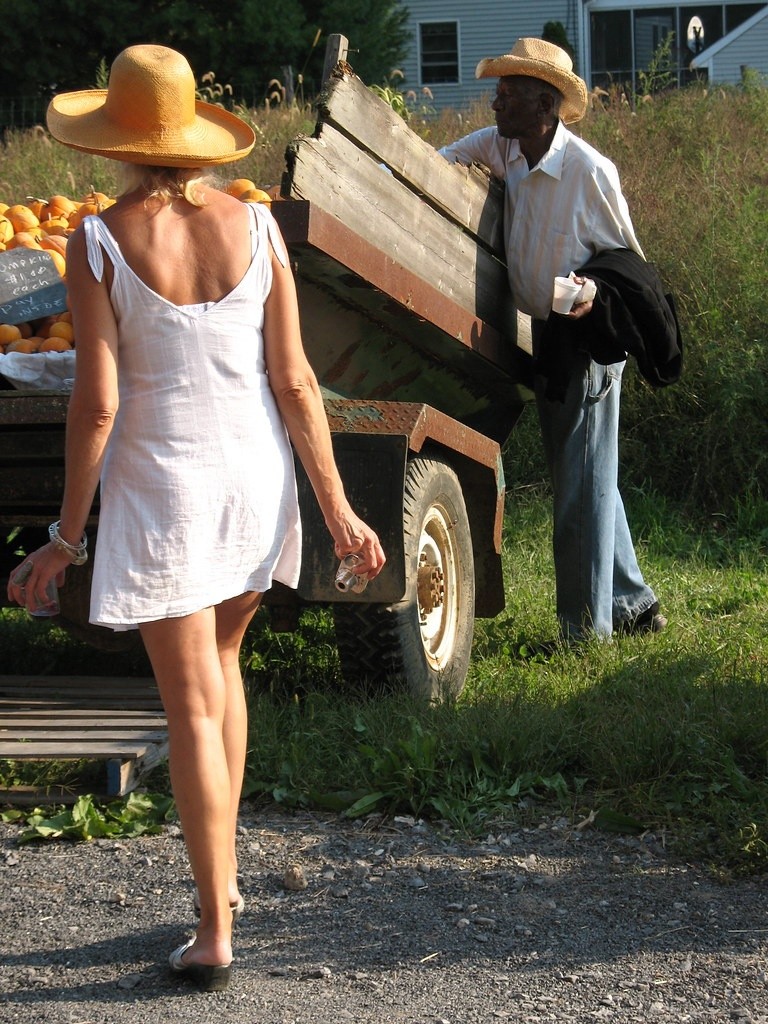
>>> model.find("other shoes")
[530,641,562,662]
[633,607,671,636]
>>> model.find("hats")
[46,44,257,168]
[472,37,589,127]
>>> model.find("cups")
[553,276,582,314]
[22,574,61,616]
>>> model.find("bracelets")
[48,520,88,565]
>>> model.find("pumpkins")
[0,179,284,353]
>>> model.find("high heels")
[192,886,246,928]
[168,940,232,995]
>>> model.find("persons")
[380,37,669,660]
[8,44,385,991]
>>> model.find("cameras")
[334,553,369,594]
